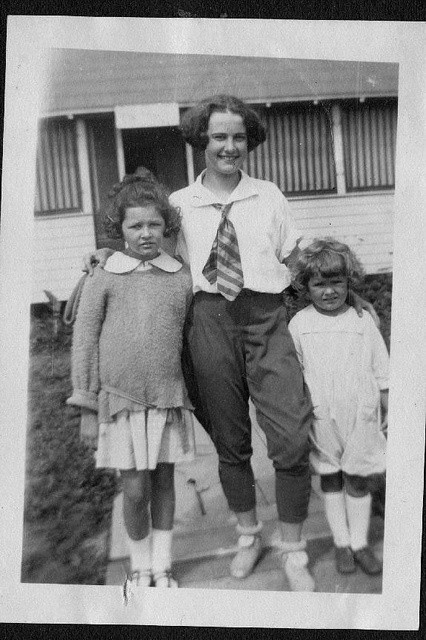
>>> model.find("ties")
[200,203,244,302]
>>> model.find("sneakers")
[278,537,314,592]
[230,521,262,578]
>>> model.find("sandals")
[125,568,150,587]
[151,568,177,588]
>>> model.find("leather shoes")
[352,547,382,573]
[332,545,354,573]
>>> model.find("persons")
[81,96,380,593]
[288,240,391,576]
[65,169,192,591]
